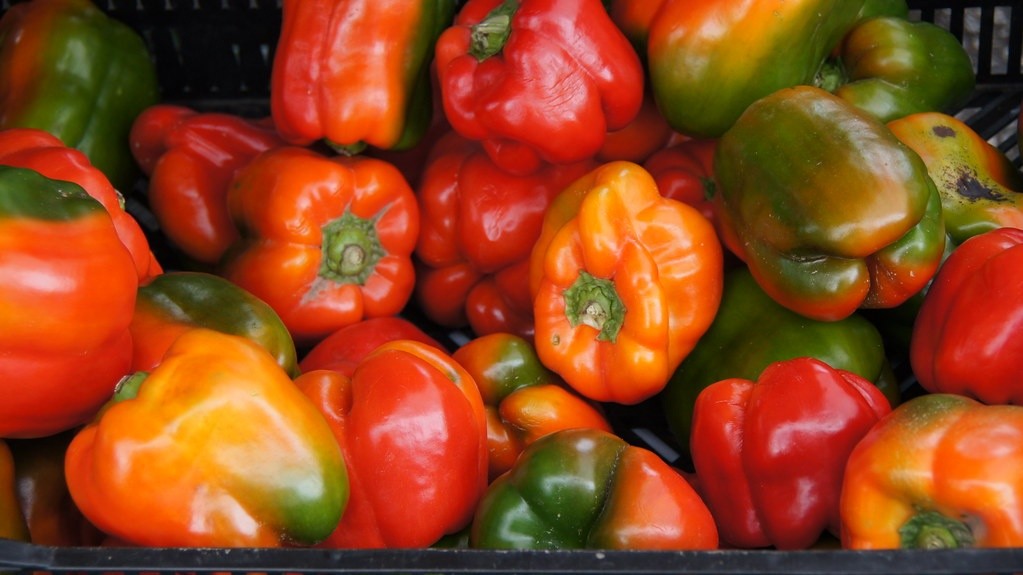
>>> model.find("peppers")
[0,0,1023,553]
